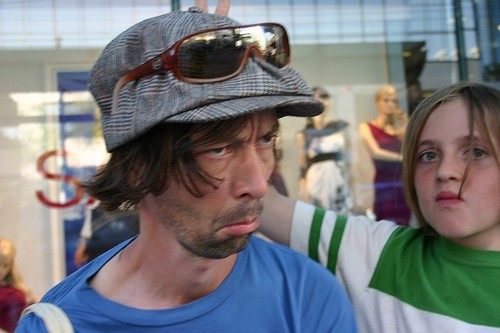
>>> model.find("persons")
[0,238,38,333]
[295,86,353,216]
[14,6,360,333]
[358,84,412,226]
[258,82,500,333]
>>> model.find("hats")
[87,6,324,153]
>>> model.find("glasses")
[110,23,291,115]
